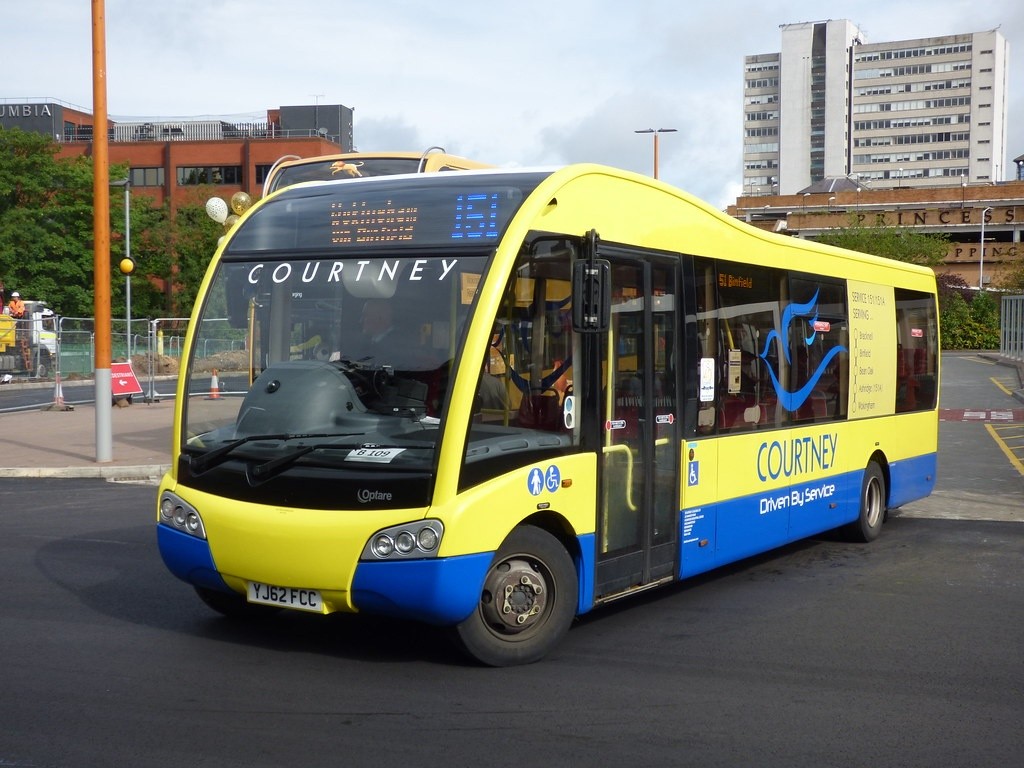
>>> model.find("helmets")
[12,292,19,296]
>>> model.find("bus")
[221,147,666,425]
[156,164,940,669]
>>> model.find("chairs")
[898,344,928,380]
[506,386,828,447]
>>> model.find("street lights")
[827,196,836,211]
[960,173,964,187]
[857,188,861,209]
[899,168,903,189]
[979,207,994,291]
[803,192,811,212]
[962,182,967,207]
[751,180,757,197]
[770,176,778,196]
[856,173,860,189]
[757,188,760,196]
[635,128,678,181]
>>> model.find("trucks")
[0,301,58,379]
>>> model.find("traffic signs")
[111,362,143,397]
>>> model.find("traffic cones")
[203,368,226,400]
[53,372,64,405]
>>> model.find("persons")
[349,297,414,403]
[480,370,513,422]
[7,291,26,318]
